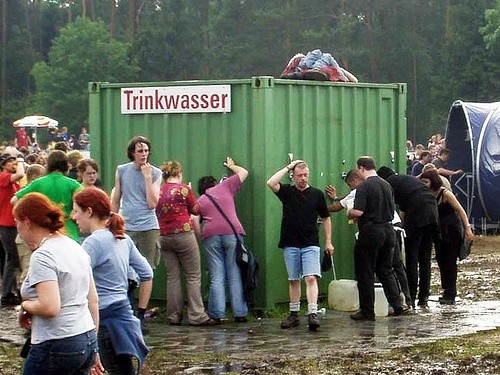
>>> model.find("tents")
[443,99,500,236]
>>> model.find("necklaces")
[38,232,54,247]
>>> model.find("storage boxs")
[87,76,408,315]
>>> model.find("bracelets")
[20,303,29,315]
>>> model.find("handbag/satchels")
[457,214,474,261]
[236,238,251,268]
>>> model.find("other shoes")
[350,312,376,321]
[1,289,23,305]
[389,298,457,314]
[201,318,220,325]
[139,320,149,335]
[235,316,248,323]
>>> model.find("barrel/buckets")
[374,283,388,316]
[327,279,360,312]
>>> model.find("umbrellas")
[12,115,58,144]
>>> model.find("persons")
[377,165,438,308]
[267,159,335,330]
[325,169,414,314]
[405,134,463,185]
[347,156,403,321]
[418,171,473,305]
[13,125,90,152]
[11,192,105,375]
[110,135,163,335]
[154,160,214,327]
[0,139,101,309]
[70,188,154,375]
[190,158,251,323]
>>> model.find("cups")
[16,158,24,175]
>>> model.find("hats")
[422,162,436,171]
[0,153,16,168]
[416,144,425,149]
[321,250,332,271]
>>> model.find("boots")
[308,313,320,330]
[280,311,301,328]
